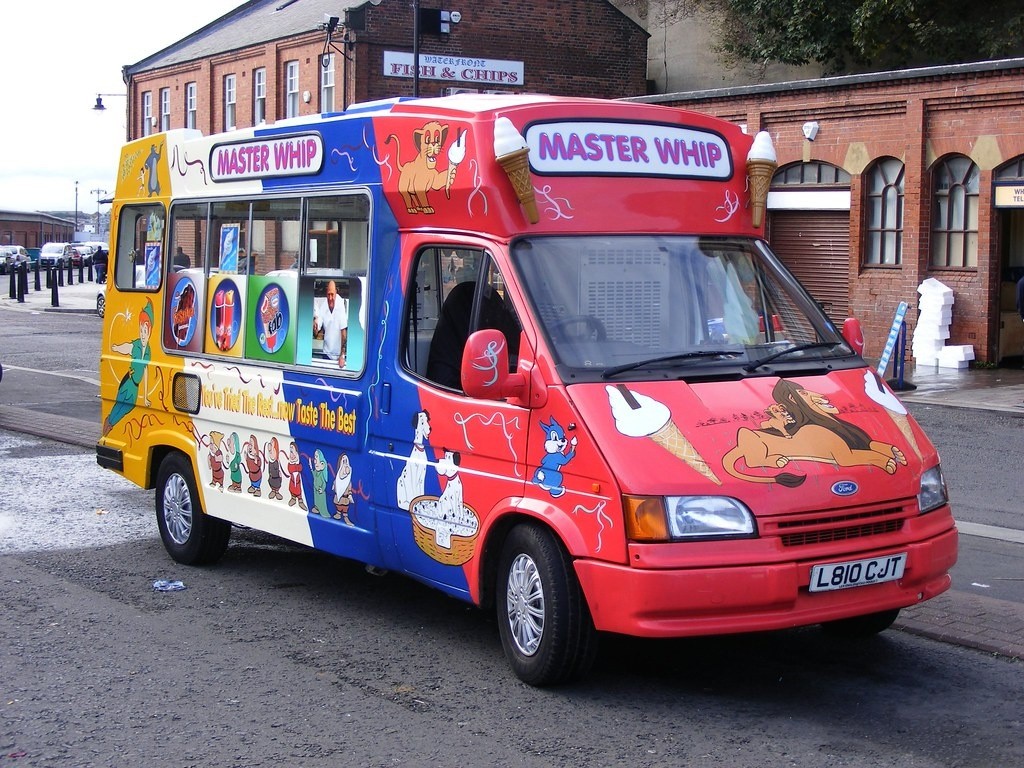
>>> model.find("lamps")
[91,93,127,110]
[802,121,818,141]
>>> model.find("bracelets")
[340,354,345,359]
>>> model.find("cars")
[0,240,109,274]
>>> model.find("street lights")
[91,188,108,235]
[74,180,80,231]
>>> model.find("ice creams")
[746,131,777,228]
[492,116,540,224]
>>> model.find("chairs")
[424,282,520,388]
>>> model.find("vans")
[96,93,962,690]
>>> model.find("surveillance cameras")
[325,13,340,23]
[317,24,324,31]
[337,25,343,32]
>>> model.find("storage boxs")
[910,277,975,369]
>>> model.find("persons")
[313,281,347,368]
[92,246,108,284]
[173,246,190,272]
[135,248,144,265]
